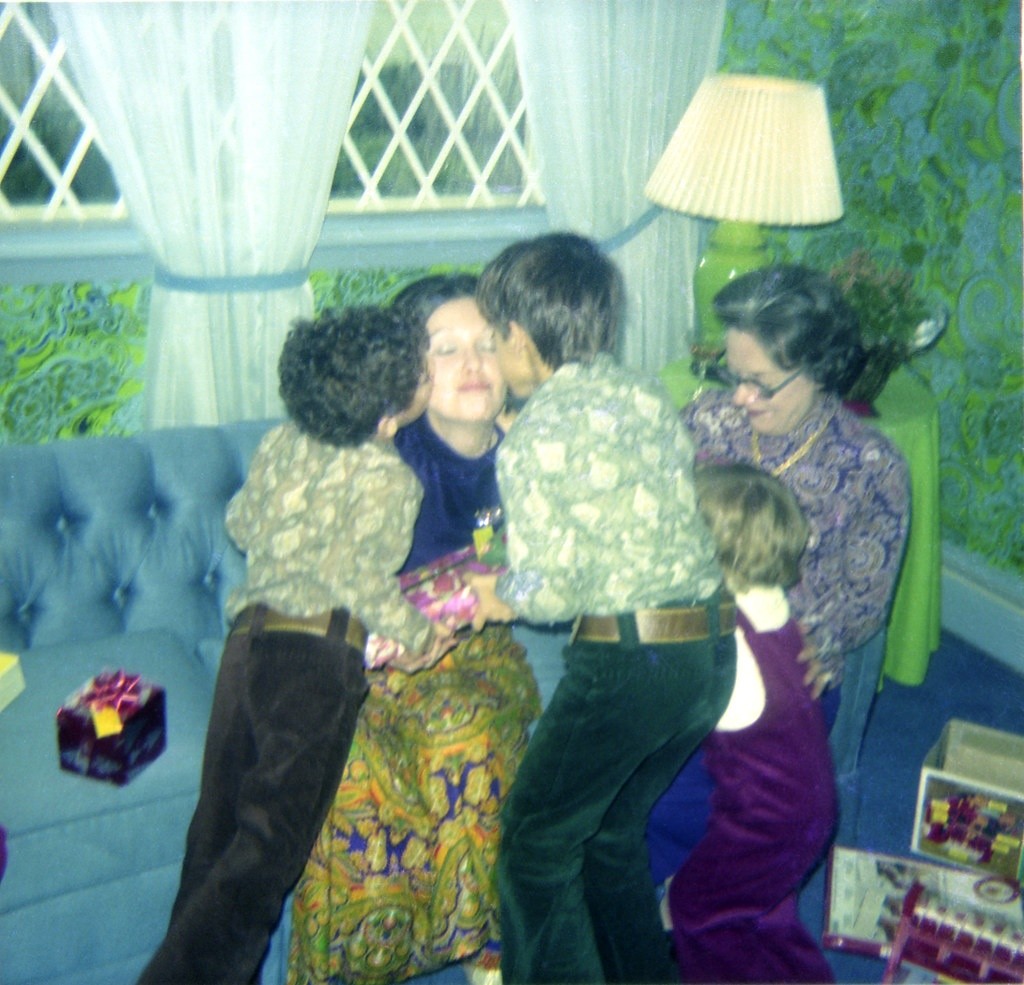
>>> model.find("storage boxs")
[57,670,166,786]
[823,719,1024,985]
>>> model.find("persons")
[669,459,842,984]
[286,273,541,985]
[457,225,740,984]
[645,265,910,886]
[136,306,434,985]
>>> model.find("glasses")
[705,346,810,399]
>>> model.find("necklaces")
[749,411,834,478]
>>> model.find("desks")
[666,363,944,691]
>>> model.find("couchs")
[0,418,578,985]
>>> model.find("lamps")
[646,72,846,377]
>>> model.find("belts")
[231,602,369,655]
[568,602,738,646]
